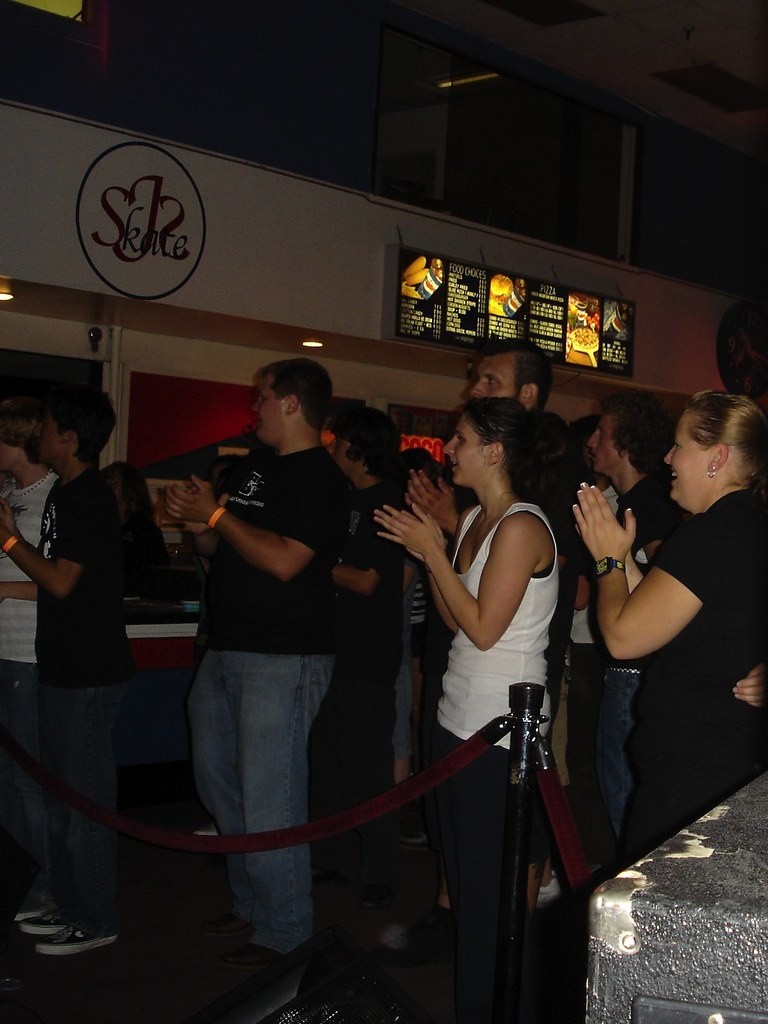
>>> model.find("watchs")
[593,556,626,576]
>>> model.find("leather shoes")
[201,913,242,937]
[219,947,249,968]
[311,859,351,887]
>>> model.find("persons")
[163,356,354,1024]
[0,338,768,1023]
[373,398,558,1024]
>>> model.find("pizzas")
[572,329,598,349]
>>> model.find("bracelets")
[1,535,20,552]
[207,507,227,528]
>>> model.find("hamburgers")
[491,274,513,308]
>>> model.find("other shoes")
[368,919,455,969]
[13,891,118,957]
[354,887,394,909]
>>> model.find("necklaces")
[10,468,53,497]
